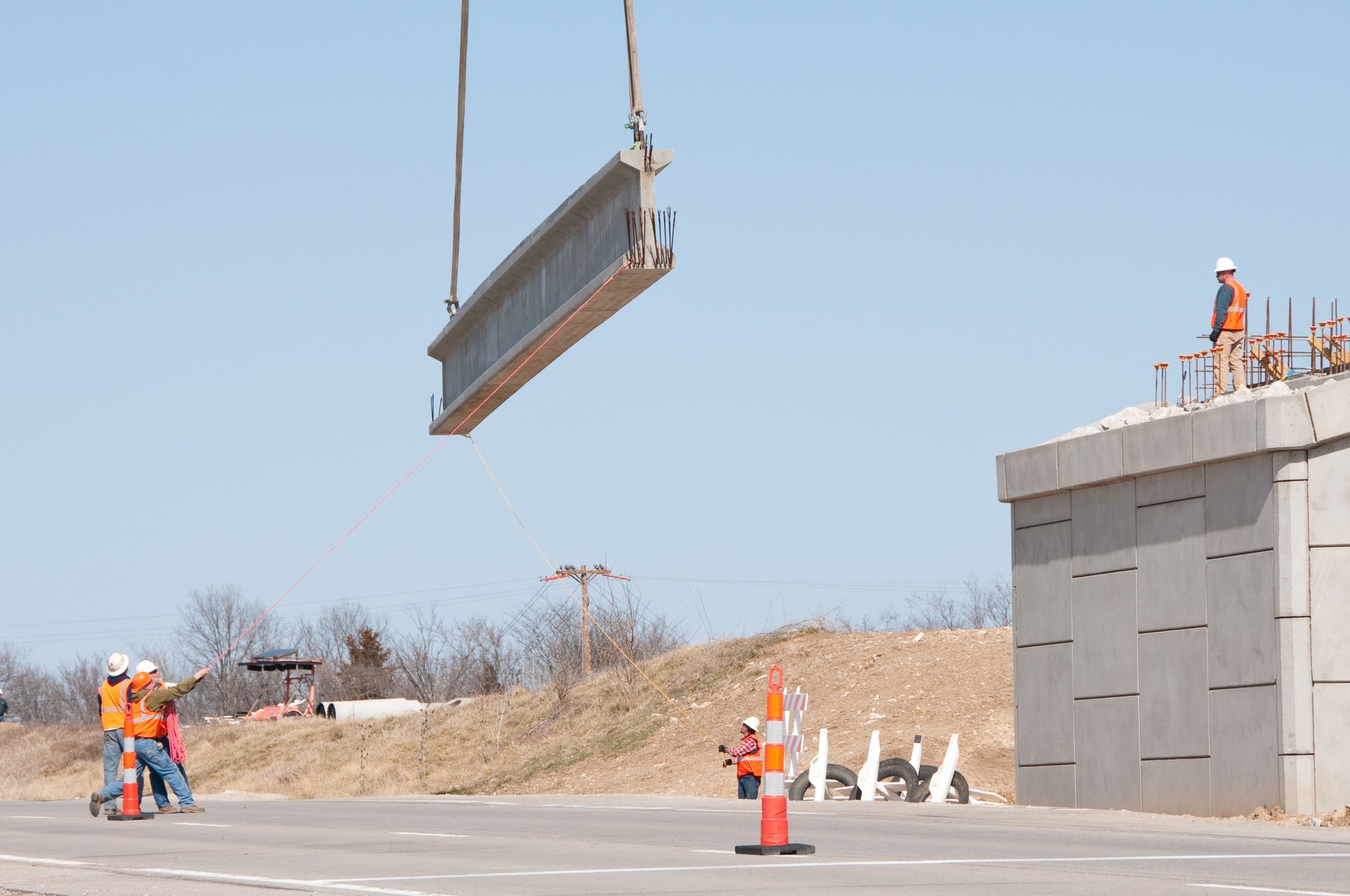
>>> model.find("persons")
[0,690,9,722]
[1209,257,1246,399]
[719,717,763,799]
[90,653,210,817]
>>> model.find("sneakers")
[159,804,177,813]
[180,805,205,813]
[104,807,122,815]
[89,791,102,817]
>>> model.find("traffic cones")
[107,703,155,821]
[735,663,816,856]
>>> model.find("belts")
[134,737,154,740]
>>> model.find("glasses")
[1216,270,1229,276]
[151,669,157,674]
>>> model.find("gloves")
[1209,330,1220,342]
[718,745,728,753]
[723,759,732,768]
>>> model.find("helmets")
[742,716,760,732]
[1214,257,1237,271]
[0,689,3,694]
[136,660,158,673]
[107,653,129,676]
[131,672,152,693]
